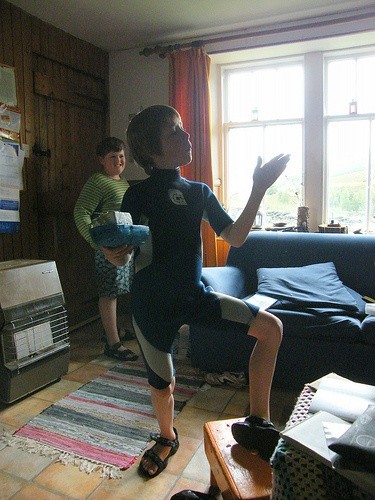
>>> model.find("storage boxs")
[270,382,375,500]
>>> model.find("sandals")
[232,415,280,463]
[139,428,179,479]
[101,326,136,342]
[104,342,138,361]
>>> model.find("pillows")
[256,261,359,318]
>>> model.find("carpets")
[1,353,212,481]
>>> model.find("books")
[278,409,352,467]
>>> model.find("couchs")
[189,231,374,392]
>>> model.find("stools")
[203,413,274,500]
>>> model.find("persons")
[75,137,138,361]
[101,105,291,480]
